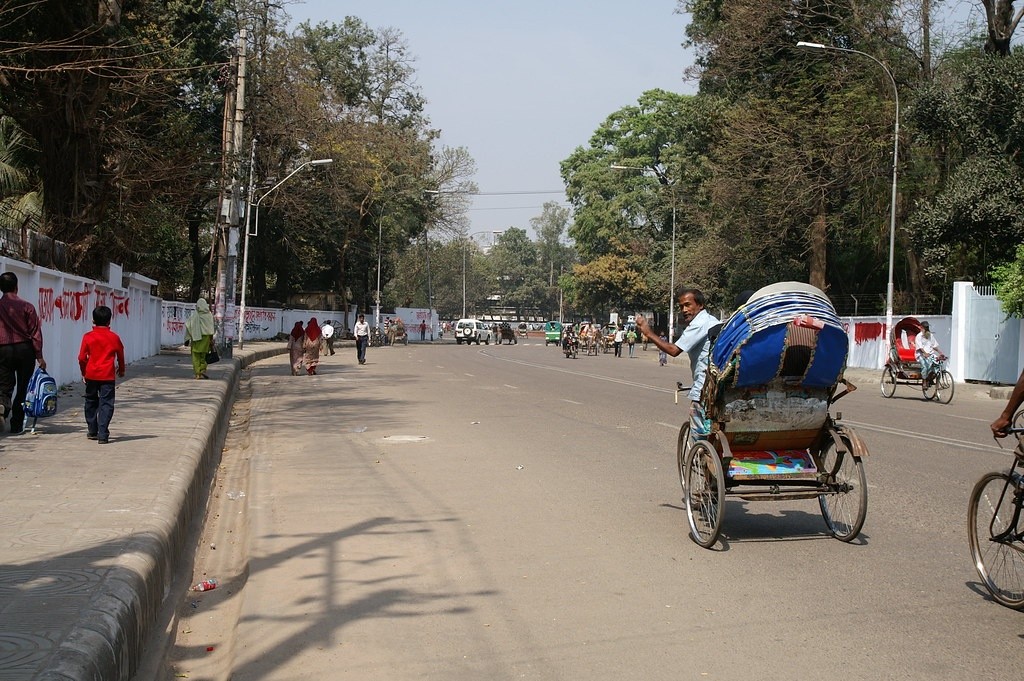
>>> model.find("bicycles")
[966,427,1024,610]
[879,317,955,404]
[1013,410,1024,441]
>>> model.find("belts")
[357,335,367,337]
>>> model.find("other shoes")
[201,371,209,379]
[192,374,200,380]
[0,404,6,433]
[86,432,98,440]
[10,429,24,436]
[98,440,109,444]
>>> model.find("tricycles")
[389,331,409,346]
[579,321,617,355]
[668,281,870,549]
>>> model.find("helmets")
[567,325,573,330]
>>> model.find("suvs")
[455,318,494,345]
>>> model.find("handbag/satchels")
[205,343,220,364]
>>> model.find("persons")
[183,298,214,379]
[613,326,624,357]
[442,321,451,333]
[304,317,322,376]
[627,327,637,358]
[518,324,526,329]
[552,324,555,329]
[640,331,648,351]
[354,314,371,365]
[562,322,610,353]
[914,321,946,390]
[383,316,392,346]
[0,272,46,436]
[78,306,125,444]
[419,320,426,341]
[492,324,501,345]
[287,321,305,376]
[658,331,667,365]
[322,320,335,356]
[708,290,756,372]
[990,370,1024,439]
[636,288,722,512]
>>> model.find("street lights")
[375,189,439,335]
[610,164,676,345]
[794,41,900,360]
[462,230,504,321]
[238,138,335,352]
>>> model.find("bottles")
[194,579,218,592]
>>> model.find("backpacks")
[20,365,58,419]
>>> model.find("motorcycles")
[563,335,579,359]
[545,321,564,345]
